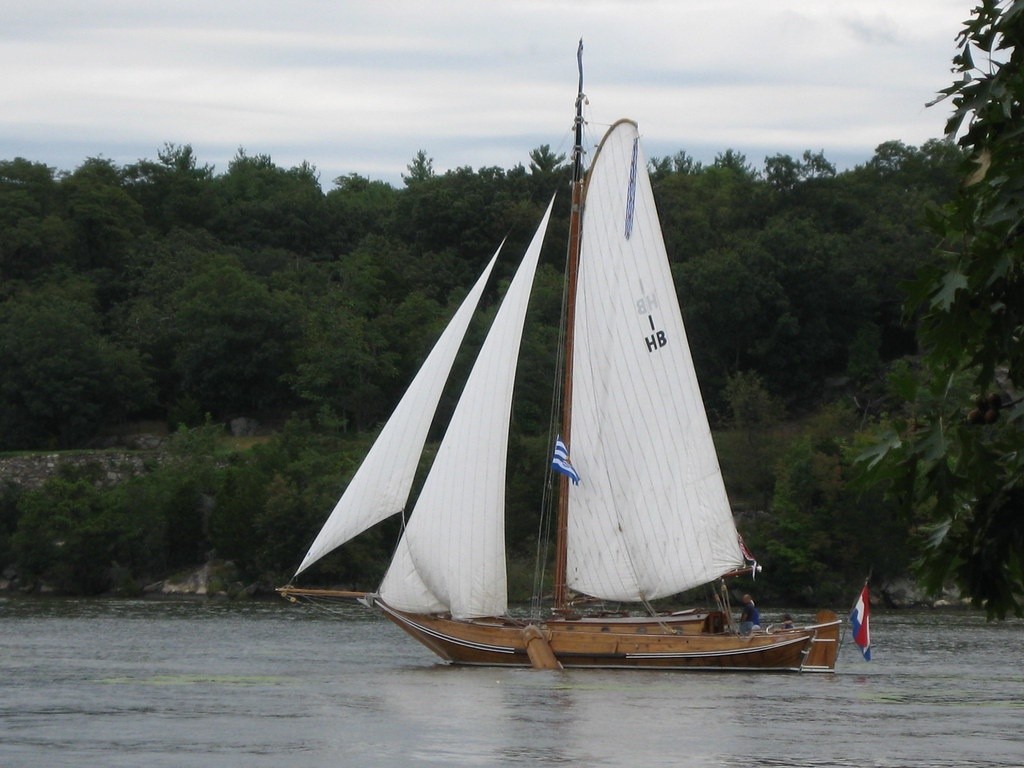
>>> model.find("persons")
[779,614,793,629]
[738,594,759,636]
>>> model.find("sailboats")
[275,34,874,672]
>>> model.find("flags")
[550,433,581,486]
[849,585,871,663]
